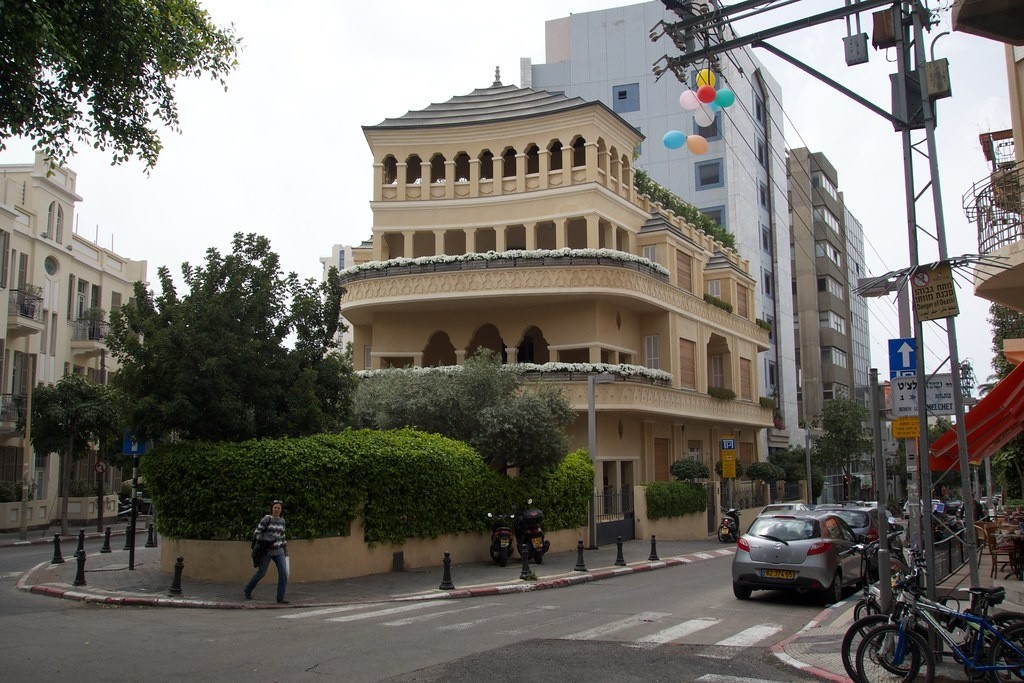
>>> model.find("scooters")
[510,498,550,565]
[488,512,515,568]
[112,491,141,518]
[718,506,743,543]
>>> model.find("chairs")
[973,507,1024,580]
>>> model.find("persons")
[244,500,291,604]
[960,494,985,553]
[1004,507,1024,537]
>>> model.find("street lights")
[858,272,927,590]
[587,372,614,549]
[805,434,823,505]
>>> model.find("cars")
[863,494,1003,524]
[732,510,875,609]
[760,502,810,514]
[812,507,904,578]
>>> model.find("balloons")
[662,69,736,153]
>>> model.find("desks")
[990,533,1024,581]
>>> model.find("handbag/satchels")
[284,556,290,578]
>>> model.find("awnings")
[931,360,1024,472]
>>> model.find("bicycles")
[841,529,1024,683]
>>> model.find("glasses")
[274,500,283,504]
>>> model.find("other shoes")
[277,599,289,603]
[245,592,252,599]
